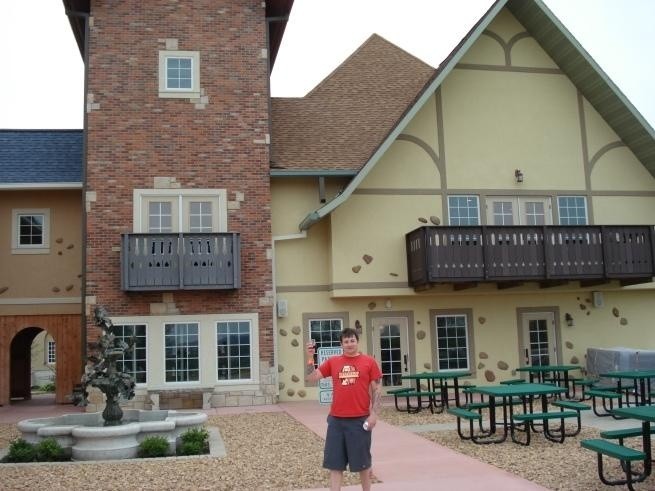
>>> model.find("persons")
[306,327,383,491]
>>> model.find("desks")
[599,371,655,408]
[516,365,581,402]
[402,372,471,412]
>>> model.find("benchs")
[387,378,655,491]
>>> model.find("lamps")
[355,320,361,334]
[566,312,573,326]
[515,168,524,182]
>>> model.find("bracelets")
[306,360,314,365]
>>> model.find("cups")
[306,339,315,354]
